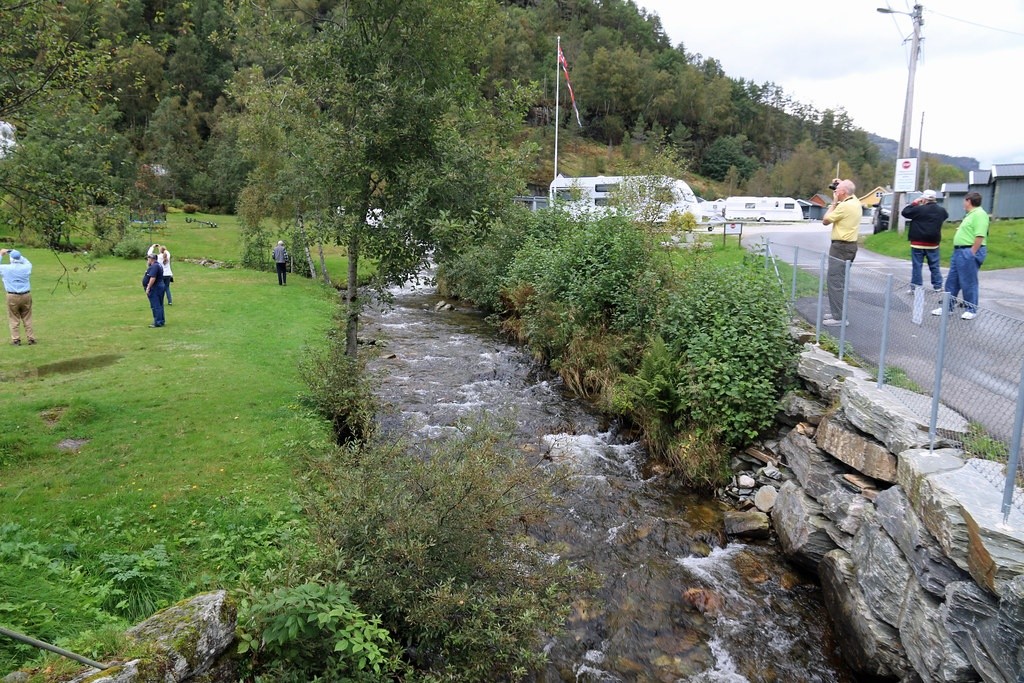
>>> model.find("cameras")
[7,250,12,253]
[829,182,838,190]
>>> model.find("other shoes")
[168,302,172,306]
[28,340,37,345]
[9,340,21,346]
[279,280,282,285]
[907,289,915,294]
[283,283,287,286]
[148,324,157,328]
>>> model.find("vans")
[550,175,702,225]
[725,196,805,224]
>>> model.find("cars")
[872,190,933,234]
[694,194,726,218]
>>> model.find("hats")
[147,254,157,260]
[921,190,936,201]
[278,241,285,245]
[10,249,20,260]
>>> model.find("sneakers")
[824,314,834,319]
[961,311,975,320]
[823,317,849,327]
[931,307,954,316]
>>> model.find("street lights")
[876,3,924,236]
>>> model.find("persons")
[900,189,950,303]
[271,240,289,286]
[821,177,863,326]
[141,243,175,328]
[930,192,990,321]
[0,249,36,344]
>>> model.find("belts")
[954,245,984,249]
[8,291,30,294]
[832,240,857,244]
